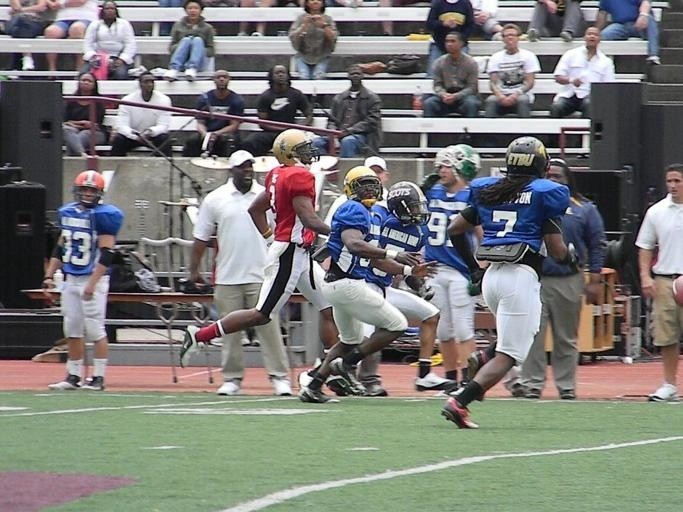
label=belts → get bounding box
[654,274,680,279]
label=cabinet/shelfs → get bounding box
[546,268,615,363]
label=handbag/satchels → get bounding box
[386,54,421,74]
[9,10,44,37]
[355,61,386,74]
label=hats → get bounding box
[364,155,386,172]
[228,149,255,170]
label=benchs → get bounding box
[0,0,668,157]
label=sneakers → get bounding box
[559,389,575,400]
[46,374,82,390]
[178,325,205,368]
[559,31,572,42]
[645,55,661,65]
[81,376,104,392]
[647,383,680,405]
[22,56,35,70]
[527,28,535,42]
[216,382,239,396]
[440,397,479,429]
[414,350,541,402]
[274,380,292,396]
[296,356,387,404]
[183,67,196,81]
[162,69,178,81]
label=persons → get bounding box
[0,0,682,428]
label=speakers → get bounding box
[568,82,683,275]
[0,80,62,208]
[0,182,47,309]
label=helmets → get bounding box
[272,128,320,167]
[504,136,550,179]
[386,180,431,226]
[433,143,481,182]
[71,170,104,205]
[342,165,383,209]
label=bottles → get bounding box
[413,85,423,110]
[56,267,62,288]
